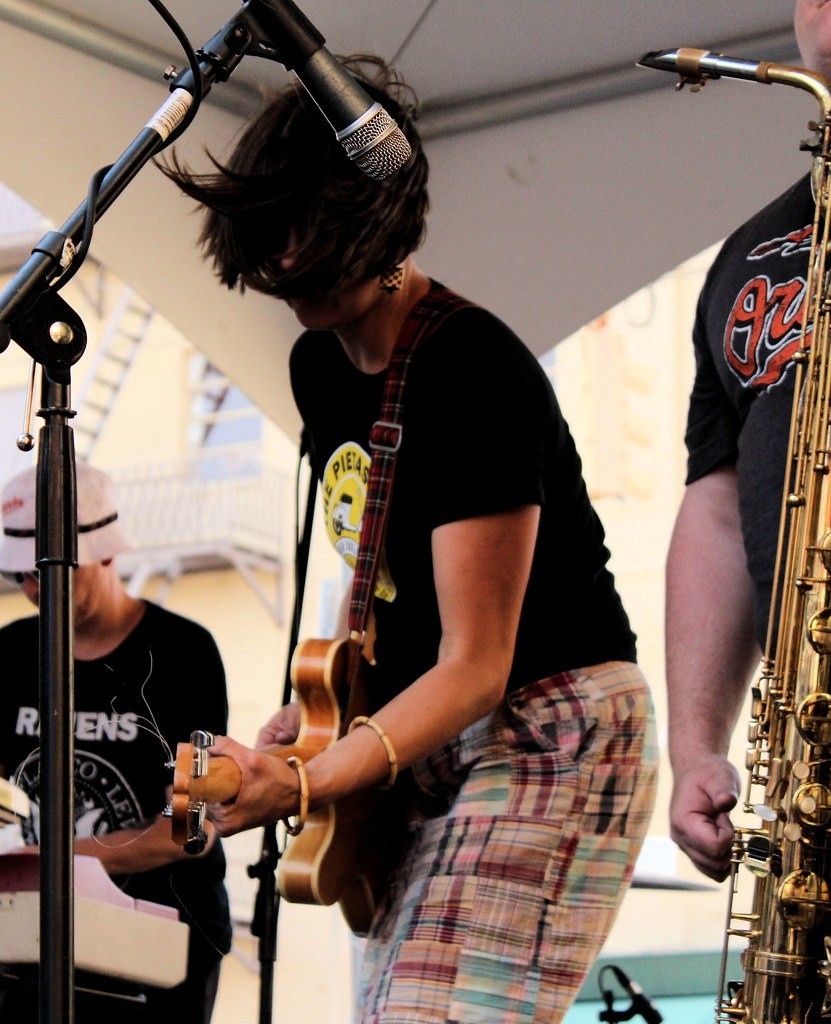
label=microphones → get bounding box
[611,964,663,1024]
[242,0,412,180]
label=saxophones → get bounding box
[636,45,831,1024]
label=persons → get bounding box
[664,1,830,883]
[146,52,658,1024]
[1,461,233,1024]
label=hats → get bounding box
[0,465,125,574]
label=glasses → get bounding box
[13,570,40,584]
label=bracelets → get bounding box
[347,716,398,791]
[282,757,309,836]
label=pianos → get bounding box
[0,779,198,1005]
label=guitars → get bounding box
[167,635,388,937]
